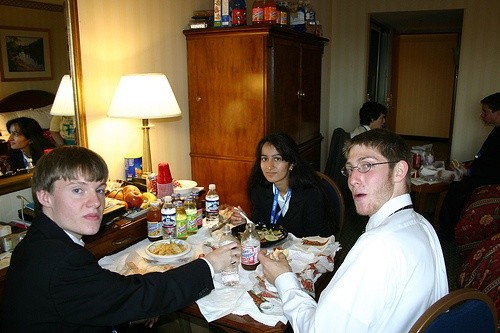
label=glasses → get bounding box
[341,159,400,177]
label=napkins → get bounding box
[410,178,442,186]
[195,287,246,323]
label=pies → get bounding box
[450,159,460,169]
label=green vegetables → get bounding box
[254,227,282,235]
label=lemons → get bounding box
[264,235,278,241]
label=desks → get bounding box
[407,159,470,233]
[98,217,343,333]
[0,190,208,302]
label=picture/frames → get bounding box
[0,26,54,82]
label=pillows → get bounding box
[0,103,54,142]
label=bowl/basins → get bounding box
[173,179,197,194]
[231,223,288,248]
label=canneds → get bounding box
[413,153,422,169]
[146,176,157,196]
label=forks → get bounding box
[234,207,259,226]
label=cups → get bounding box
[156,162,173,199]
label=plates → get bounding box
[145,239,192,258]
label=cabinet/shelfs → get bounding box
[182,23,330,230]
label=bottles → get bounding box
[251,0,315,33]
[205,184,220,225]
[184,197,198,236]
[241,223,261,271]
[190,192,202,229]
[218,225,239,286]
[231,0,247,27]
[146,193,188,241]
[425,147,434,166]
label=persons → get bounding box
[257,128,449,333]
[0,147,241,333]
[230,133,336,237]
[50,115,76,145]
[350,101,388,140]
[438,92,500,224]
[6,117,44,171]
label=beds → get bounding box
[0,89,59,156]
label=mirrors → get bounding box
[0,0,88,196]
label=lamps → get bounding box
[49,75,76,116]
[107,73,183,180]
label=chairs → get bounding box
[409,287,498,333]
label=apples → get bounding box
[108,185,144,208]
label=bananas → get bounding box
[139,192,157,209]
